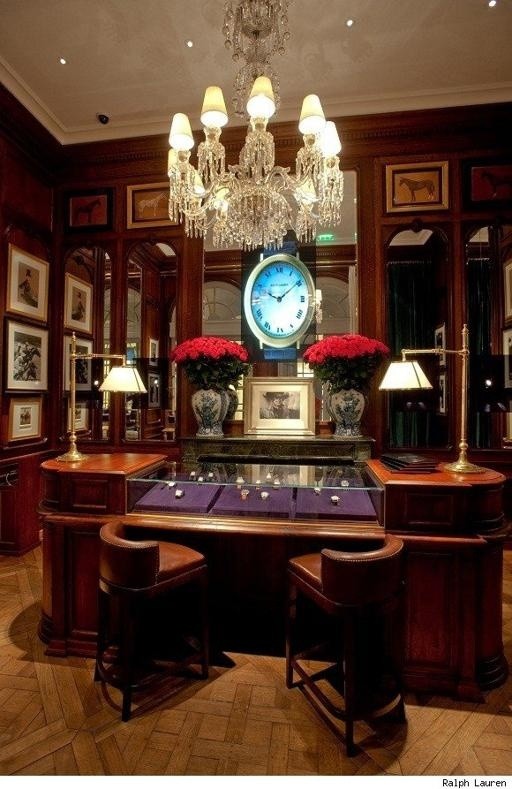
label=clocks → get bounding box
[242,251,322,353]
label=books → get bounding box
[377,452,438,474]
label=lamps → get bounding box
[164,1,346,252]
[376,321,483,477]
[53,327,150,466]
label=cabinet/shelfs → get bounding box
[39,447,508,705]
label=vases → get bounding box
[327,388,373,438]
[192,385,229,438]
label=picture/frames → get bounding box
[62,270,94,435]
[501,259,512,327]
[460,155,511,213]
[432,322,447,372]
[147,336,160,368]
[6,242,50,323]
[381,158,452,218]
[436,371,448,417]
[241,375,316,438]
[3,314,50,399]
[9,397,46,443]
[126,182,180,231]
[500,327,512,391]
[146,370,161,409]
[64,184,116,234]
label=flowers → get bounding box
[301,331,393,395]
[169,335,251,394]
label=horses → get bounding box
[135,191,168,218]
[74,199,101,225]
[22,347,41,365]
[398,177,435,201]
[480,171,512,194]
[13,362,38,381]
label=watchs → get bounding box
[167,471,351,506]
[242,238,323,362]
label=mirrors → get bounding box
[388,227,453,451]
[62,242,111,440]
[465,224,512,450]
[127,240,179,441]
[198,171,357,344]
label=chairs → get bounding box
[161,410,176,441]
[282,533,416,762]
[89,516,215,722]
